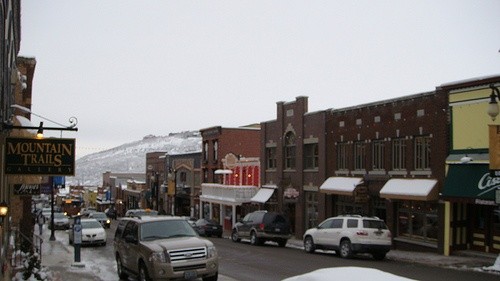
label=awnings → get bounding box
[10,114,50,185]
[379,178,439,201]
[251,187,277,203]
[319,176,365,196]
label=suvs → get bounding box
[230,209,293,248]
[42,208,51,219]
[113,213,218,281]
[303,214,393,258]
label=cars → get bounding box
[88,212,110,228]
[68,218,107,247]
[182,216,196,226]
[125,208,158,215]
[72,206,96,223]
[46,213,70,230]
[191,218,223,237]
